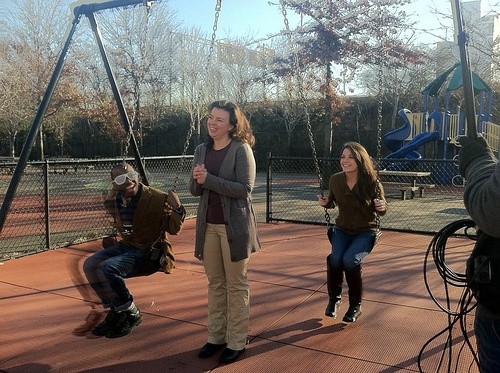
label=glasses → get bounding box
[110,172,140,185]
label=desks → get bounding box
[48,158,94,174]
[377,170,431,199]
[0,163,30,175]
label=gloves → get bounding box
[453,132,492,178]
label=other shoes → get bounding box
[105,308,143,337]
[198,343,227,359]
[219,348,246,364]
[325,299,341,317]
[91,310,122,337]
[343,305,361,322]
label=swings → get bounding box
[278,0,385,250]
[111,1,222,274]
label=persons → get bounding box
[187,99,262,363]
[82,162,186,338]
[458,130,500,373]
[319,142,388,322]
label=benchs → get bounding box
[380,182,436,189]
[380,187,419,191]
[50,165,94,170]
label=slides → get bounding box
[381,107,441,170]
